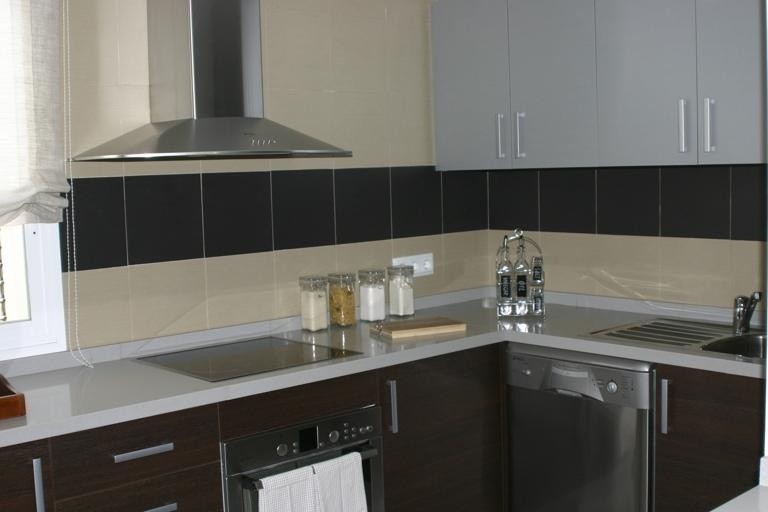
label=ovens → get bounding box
[218,404,387,512]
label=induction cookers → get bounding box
[128,335,366,384]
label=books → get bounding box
[371,315,468,339]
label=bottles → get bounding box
[296,264,417,334]
[495,235,544,315]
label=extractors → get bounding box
[64,1,354,164]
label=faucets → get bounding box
[734,291,762,333]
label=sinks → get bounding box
[700,333,766,364]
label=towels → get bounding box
[257,452,370,511]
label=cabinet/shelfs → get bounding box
[52,403,223,511]
[0,439,50,512]
[381,340,508,512]
[429,1,596,173]
[595,1,765,170]
[650,364,765,512]
